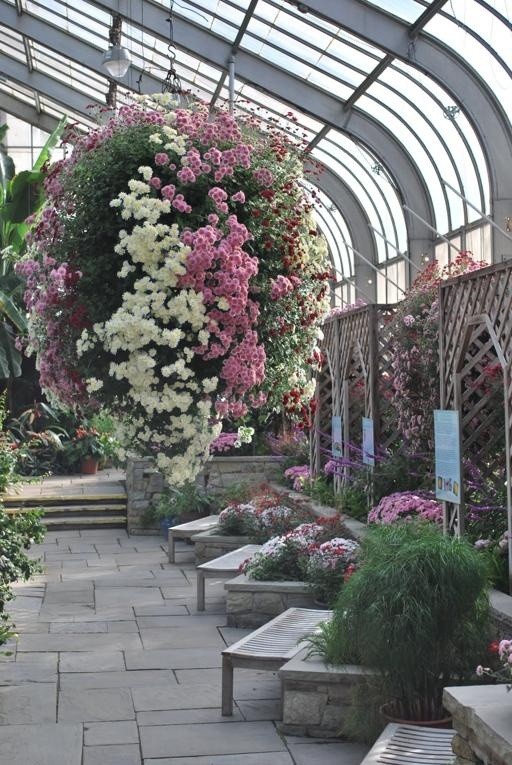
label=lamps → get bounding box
[102,40,134,78]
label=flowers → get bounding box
[11,97,340,489]
[306,534,364,598]
[70,419,107,460]
[474,634,512,683]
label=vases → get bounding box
[314,599,334,612]
[79,458,98,475]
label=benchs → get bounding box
[360,721,460,765]
[195,544,263,611]
[165,510,216,564]
[220,604,337,720]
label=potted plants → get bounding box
[137,482,222,538]
[295,520,504,747]
